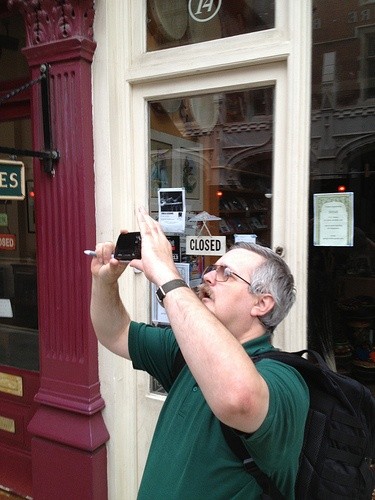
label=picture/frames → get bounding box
[150,128,178,212]
[178,137,203,212]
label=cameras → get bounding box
[114,231,141,260]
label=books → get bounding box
[219,185,270,247]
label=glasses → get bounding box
[202,265,266,296]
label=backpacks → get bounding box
[218,349,375,500]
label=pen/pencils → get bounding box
[82,249,112,264]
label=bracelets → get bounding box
[155,278,190,308]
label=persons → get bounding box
[90,209,311,499]
